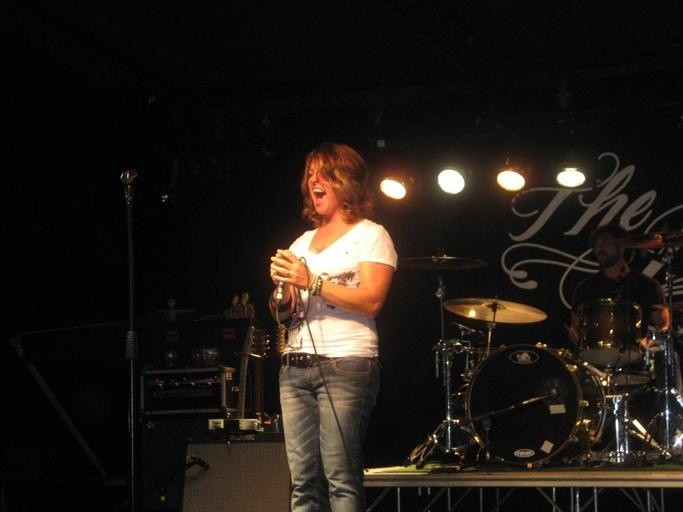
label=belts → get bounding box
[279,352,316,368]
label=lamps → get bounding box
[379,165,587,200]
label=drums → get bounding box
[608,326,658,392]
[464,342,609,465]
[617,385,683,459]
[577,297,646,367]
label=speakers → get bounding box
[138,412,236,512]
[177,429,293,512]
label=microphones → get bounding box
[547,387,560,401]
[190,454,209,469]
[457,324,484,336]
[276,248,295,304]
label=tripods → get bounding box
[601,258,682,463]
[403,272,474,469]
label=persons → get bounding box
[269,142,398,512]
[567,224,681,463]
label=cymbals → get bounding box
[442,297,548,323]
[399,255,488,273]
[612,230,683,249]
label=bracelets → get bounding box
[310,275,322,297]
